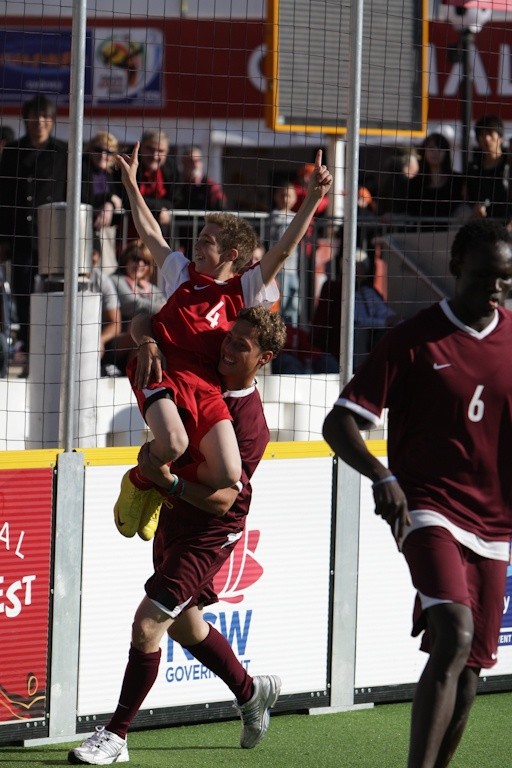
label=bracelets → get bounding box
[137,339,157,353]
[371,475,396,489]
[163,473,185,499]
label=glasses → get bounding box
[94,146,116,157]
[132,255,153,266]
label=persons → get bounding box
[65,303,287,765]
[0,94,512,379]
[114,139,333,541]
[320,217,512,768]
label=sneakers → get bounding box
[113,469,154,537]
[233,674,282,749]
[139,488,173,542]
[68,724,130,766]
